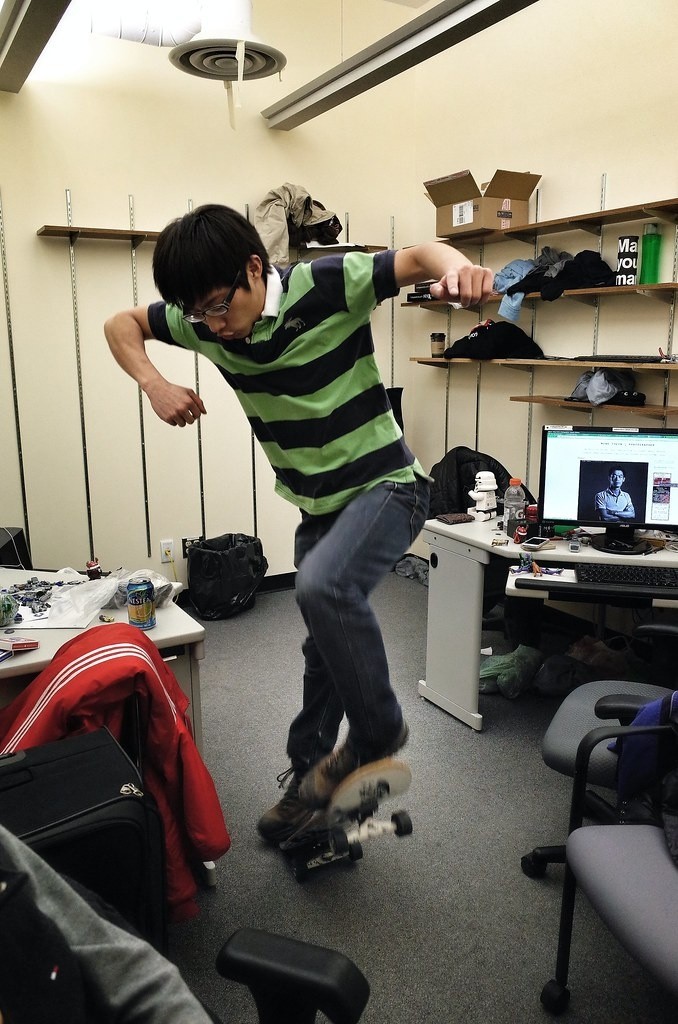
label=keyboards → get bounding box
[574,562,678,587]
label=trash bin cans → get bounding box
[189,534,260,620]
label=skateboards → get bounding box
[279,756,414,888]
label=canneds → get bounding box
[126,577,157,630]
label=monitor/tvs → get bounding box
[538,425,678,555]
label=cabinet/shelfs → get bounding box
[402,198,678,417]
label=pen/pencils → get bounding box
[549,538,568,541]
[643,546,664,556]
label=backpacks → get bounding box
[0,870,90,1024]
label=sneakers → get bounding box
[298,721,408,806]
[257,758,318,840]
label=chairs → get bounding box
[521,622,678,1012]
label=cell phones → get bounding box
[521,537,550,549]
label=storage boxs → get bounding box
[422,168,541,237]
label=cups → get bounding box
[430,332,446,358]
[615,236,639,286]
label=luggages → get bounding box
[0,728,169,960]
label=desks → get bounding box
[1,603,204,763]
[419,517,678,730]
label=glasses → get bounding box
[182,270,241,323]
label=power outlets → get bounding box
[159,536,203,562]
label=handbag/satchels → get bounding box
[479,635,634,700]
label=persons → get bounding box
[104,204,493,842]
[594,466,636,523]
[0,826,223,1024]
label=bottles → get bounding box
[502,478,525,533]
[638,222,663,285]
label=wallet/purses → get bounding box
[435,513,475,525]
